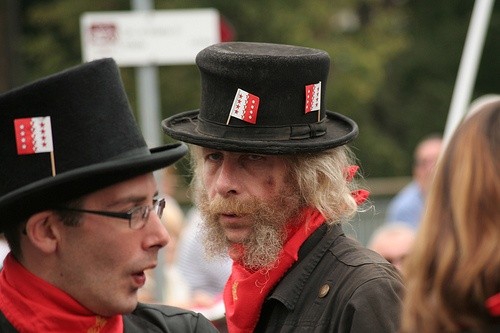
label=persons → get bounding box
[387,137,447,230]
[366,219,413,276]
[400,98,500,333]
[0,55,222,333]
[135,164,233,320]
[160,42,406,333]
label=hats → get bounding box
[0,58,188,217]
[161,40,358,154]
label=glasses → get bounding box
[26,198,164,230]
